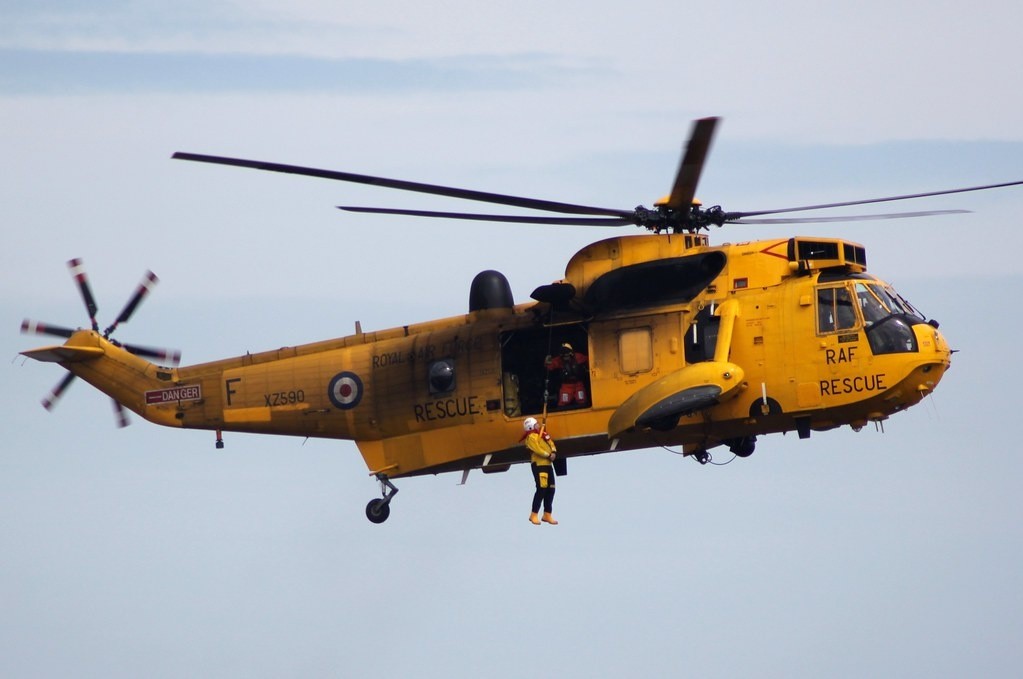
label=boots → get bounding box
[541,512,558,524]
[529,512,541,524]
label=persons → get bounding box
[545,342,588,406]
[524,417,558,525]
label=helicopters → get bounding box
[13,115,1023,526]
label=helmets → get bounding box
[562,343,572,350]
[524,417,537,432]
